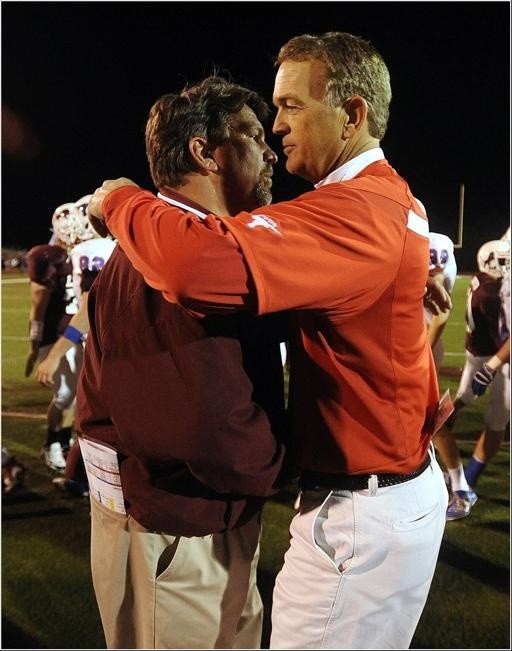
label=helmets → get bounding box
[477,240,512,279]
[51,201,77,246]
[74,194,101,240]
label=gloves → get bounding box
[471,354,503,396]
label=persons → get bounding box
[86,32,451,650]
[1,194,510,520]
[70,77,300,651]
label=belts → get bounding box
[298,450,432,492]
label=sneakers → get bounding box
[40,440,67,474]
[446,485,478,522]
[1,458,25,497]
